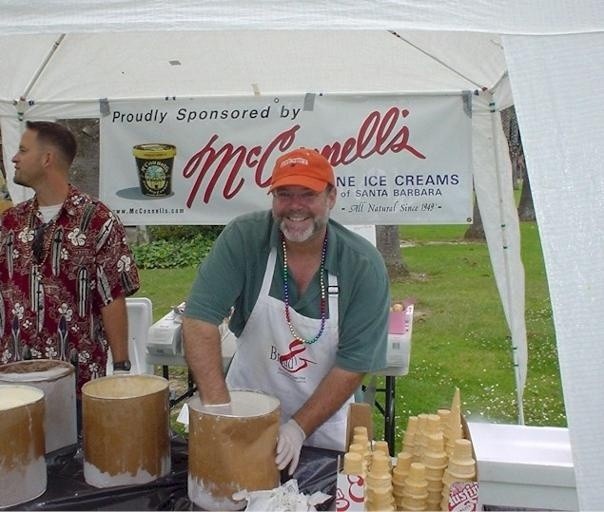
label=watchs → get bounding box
[113,360,132,371]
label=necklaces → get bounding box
[282,236,328,345]
[24,210,57,268]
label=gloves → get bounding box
[274,418,306,475]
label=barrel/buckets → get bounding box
[79,372,173,491]
[0,384,48,510]
[184,388,284,511]
[0,359,77,455]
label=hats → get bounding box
[266,149,335,195]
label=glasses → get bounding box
[31,223,44,250]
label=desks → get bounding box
[147,311,409,455]
[1,435,393,509]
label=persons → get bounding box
[183,148,391,476]
[1,120,140,438]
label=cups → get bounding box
[130,144,176,198]
